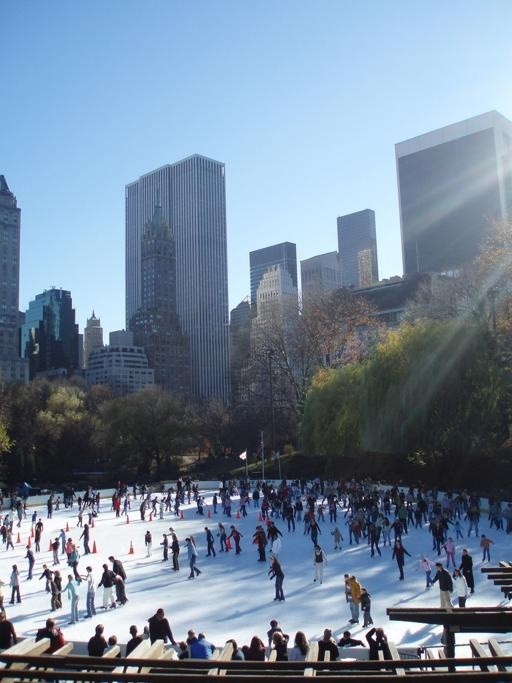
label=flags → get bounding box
[239,451,247,460]
